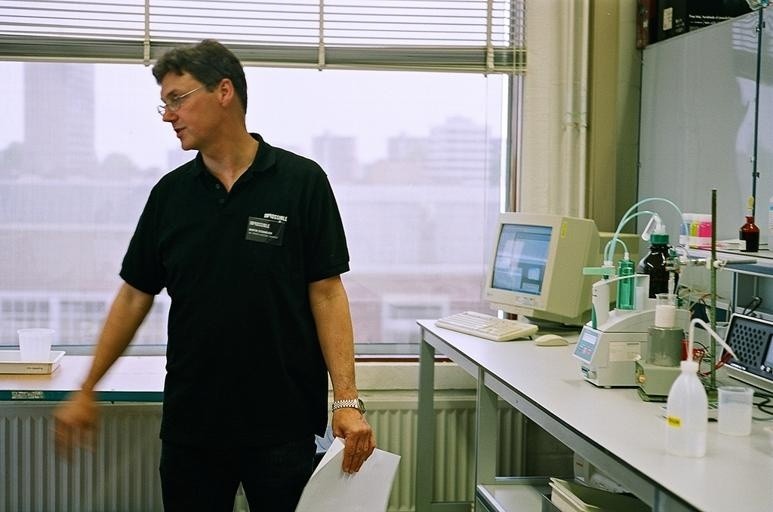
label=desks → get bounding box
[413,316,772,510]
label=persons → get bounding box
[54,38,376,512]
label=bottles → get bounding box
[616,258,635,310]
[666,360,709,458]
[767,195,773,250]
[740,196,759,252]
[679,212,712,249]
[642,233,681,299]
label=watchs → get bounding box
[332,397,367,414]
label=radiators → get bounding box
[0,395,527,512]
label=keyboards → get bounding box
[437,311,537,341]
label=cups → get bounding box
[16,330,51,360]
[653,292,677,327]
[719,387,754,438]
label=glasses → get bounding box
[157,76,232,117]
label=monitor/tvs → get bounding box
[486,208,620,329]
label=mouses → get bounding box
[536,334,568,345]
